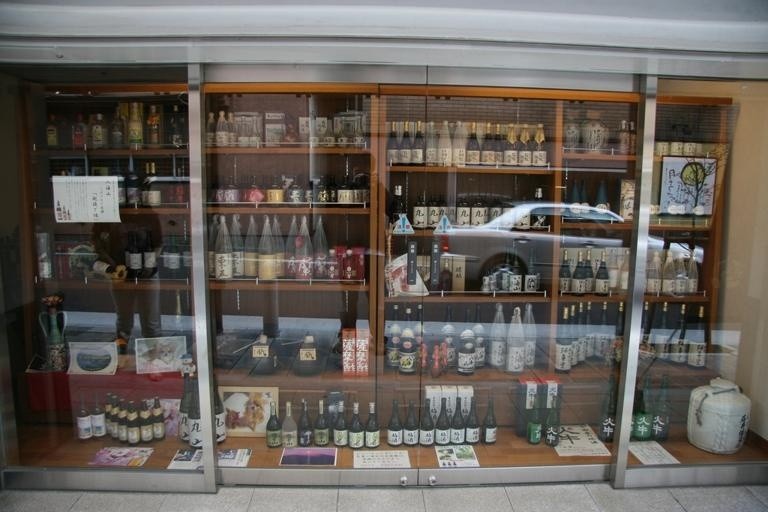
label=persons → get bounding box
[92,171,163,354]
[320,152,402,355]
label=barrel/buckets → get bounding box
[689,378,751,452]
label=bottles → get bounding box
[73,390,168,446]
[439,257,453,293]
[659,117,710,157]
[212,376,230,447]
[601,372,672,441]
[204,154,368,288]
[527,394,561,446]
[643,303,704,369]
[207,109,263,148]
[280,118,300,146]
[387,119,549,168]
[385,172,611,230]
[615,116,636,156]
[564,109,609,154]
[33,95,191,282]
[386,305,540,374]
[479,229,700,297]
[560,303,622,367]
[385,396,500,445]
[265,395,381,451]
[308,109,366,150]
[177,374,200,448]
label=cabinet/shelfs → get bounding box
[1,81,767,488]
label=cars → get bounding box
[432,204,705,292]
